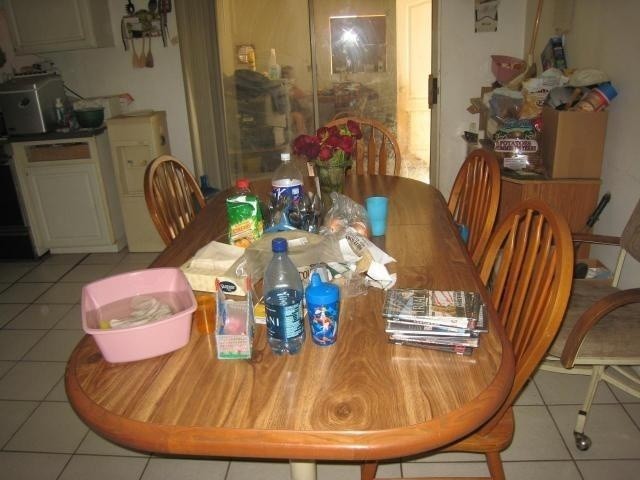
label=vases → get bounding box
[316,162,344,214]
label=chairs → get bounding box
[447,148,500,272]
[317,117,401,179]
[142,155,207,250]
[548,198,640,451]
[358,197,575,480]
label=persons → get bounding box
[282,64,311,135]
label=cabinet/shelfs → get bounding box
[1,124,128,262]
[0,0,114,57]
[496,175,599,259]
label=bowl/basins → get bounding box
[80,266,199,364]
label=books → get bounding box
[384,288,490,355]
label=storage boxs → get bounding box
[541,100,611,180]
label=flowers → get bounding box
[294,119,362,194]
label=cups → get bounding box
[194,298,217,335]
[364,195,390,237]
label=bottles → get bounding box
[268,48,281,81]
[305,273,341,347]
[263,237,306,357]
[270,153,305,233]
[225,178,261,247]
[55,97,65,123]
